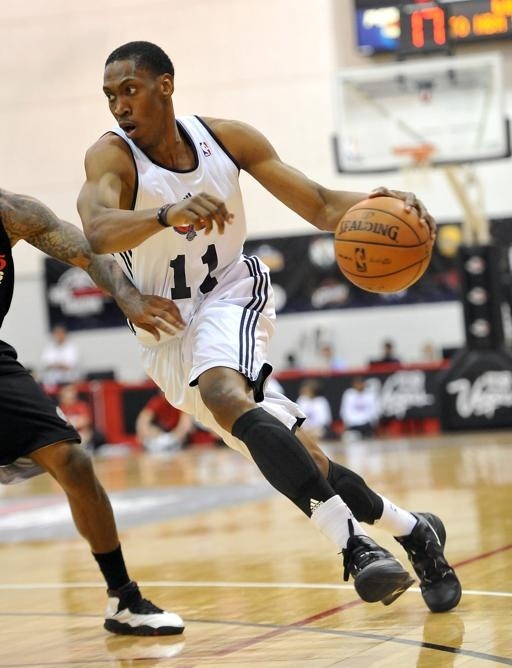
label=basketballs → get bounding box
[335,196,433,294]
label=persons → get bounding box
[33,321,199,456]
[278,330,456,443]
[0,188,192,638]
[77,40,461,614]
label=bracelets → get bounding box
[157,203,174,227]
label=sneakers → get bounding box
[397,511,461,613]
[104,580,184,636]
[342,518,415,605]
